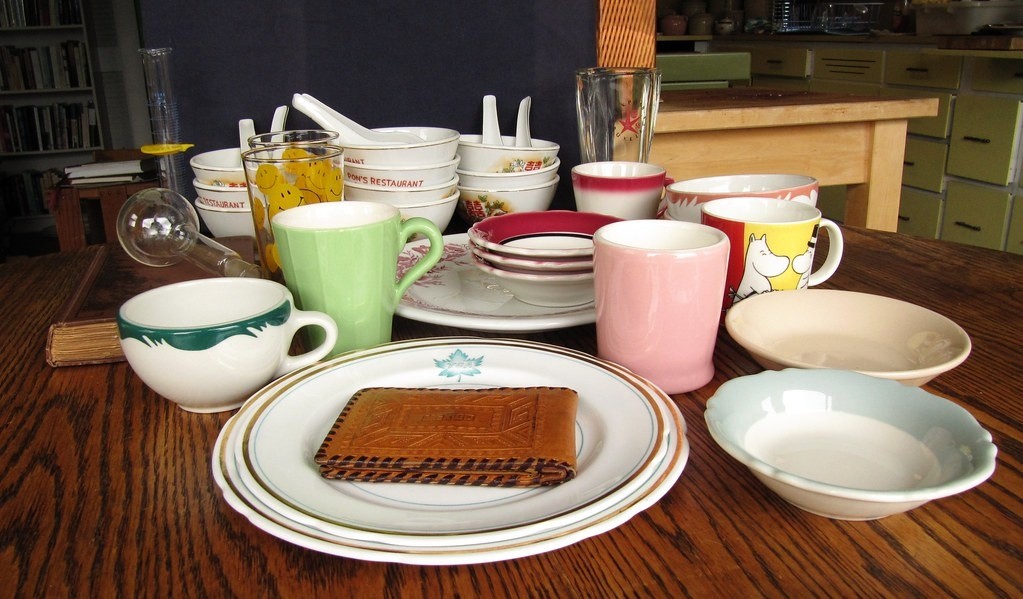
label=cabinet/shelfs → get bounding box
[714,39,1023,254]
[0,0,106,244]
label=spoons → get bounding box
[291,91,425,146]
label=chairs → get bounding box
[91,149,155,163]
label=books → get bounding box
[0,0,104,218]
[63,156,155,184]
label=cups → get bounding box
[575,66,662,165]
[116,276,337,413]
[271,200,444,361]
[701,197,844,328]
[593,219,731,396]
[571,161,676,220]
[242,131,345,281]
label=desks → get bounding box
[0,222,1023,599]
[48,180,160,251]
[651,86,941,234]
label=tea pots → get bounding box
[661,11,688,35]
[689,10,713,35]
[714,17,739,34]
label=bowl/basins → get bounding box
[190,147,317,261]
[456,133,560,225]
[327,127,461,243]
[664,175,819,224]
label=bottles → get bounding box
[116,187,271,281]
[892,0,902,33]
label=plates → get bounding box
[211,335,689,567]
[703,368,998,520]
[395,232,599,333]
[467,210,624,307]
[724,290,972,388]
